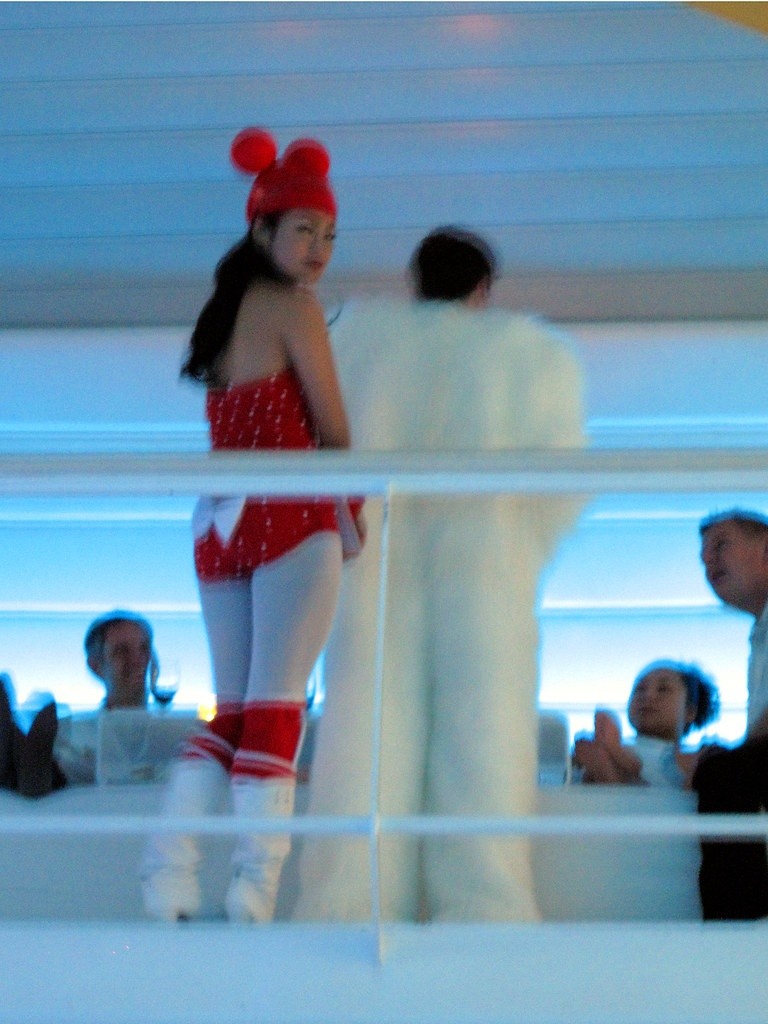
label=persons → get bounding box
[564,507,768,927]
[0,608,178,800]
[138,129,369,930]
[293,227,593,927]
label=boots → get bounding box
[223,783,295,925]
[141,759,227,926]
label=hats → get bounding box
[230,127,337,227]
